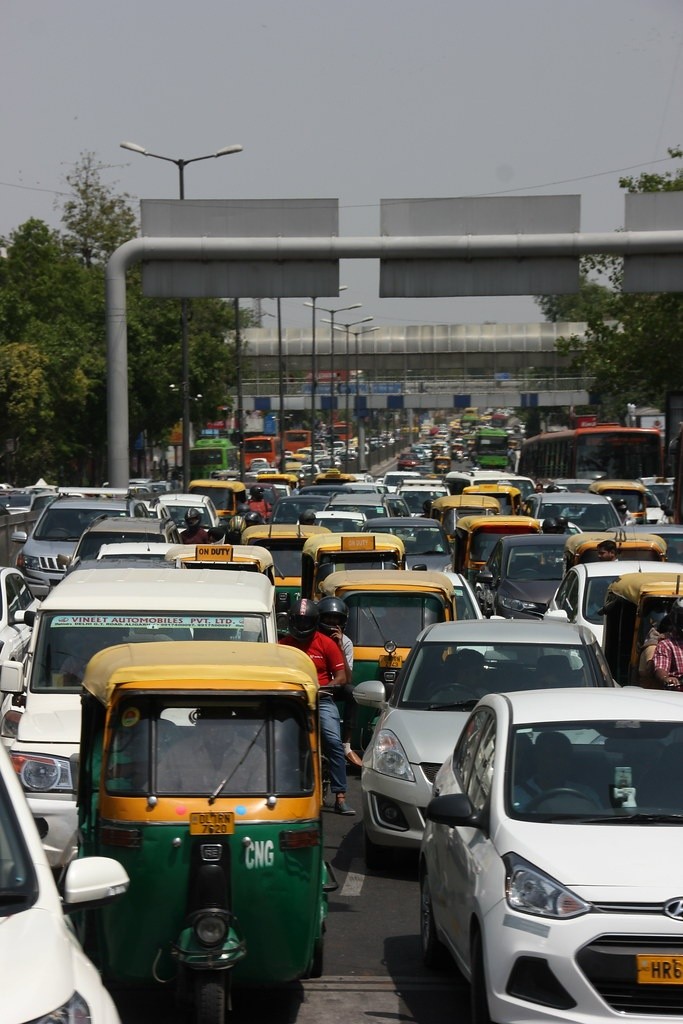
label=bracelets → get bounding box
[664,676,667,677]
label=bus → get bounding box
[326,421,354,439]
[474,428,511,469]
[236,437,282,473]
[284,430,311,452]
[188,438,240,481]
[517,427,664,482]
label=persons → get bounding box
[278,595,362,815]
[429,649,491,704]
[535,483,560,493]
[413,500,440,531]
[82,699,273,833]
[59,636,104,681]
[597,540,618,561]
[561,504,586,518]
[543,518,562,534]
[180,485,273,544]
[512,731,604,814]
[299,511,316,525]
[639,596,683,687]
[293,478,305,494]
[615,497,636,525]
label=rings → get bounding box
[669,680,671,682]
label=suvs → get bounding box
[8,491,150,599]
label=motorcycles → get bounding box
[434,455,453,474]
[71,641,326,1024]
[565,531,668,570]
[316,568,458,768]
[188,480,247,523]
[597,571,682,688]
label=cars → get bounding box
[541,562,682,671]
[424,687,683,1023]
[352,619,643,847]
[396,452,421,471]
[475,533,572,622]
[0,409,683,619]
[2,568,40,712]
[0,567,278,874]
[1,743,130,1024]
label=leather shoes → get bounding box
[344,750,363,767]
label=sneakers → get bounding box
[334,801,356,814]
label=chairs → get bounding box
[531,654,578,689]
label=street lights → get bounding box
[302,285,380,480]
[120,138,244,499]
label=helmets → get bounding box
[247,485,265,499]
[184,508,201,529]
[288,598,319,640]
[547,486,559,493]
[316,596,349,634]
[614,497,627,510]
[542,516,569,529]
[245,512,260,523]
[423,499,434,512]
[237,505,249,512]
[299,511,316,525]
[229,516,246,532]
